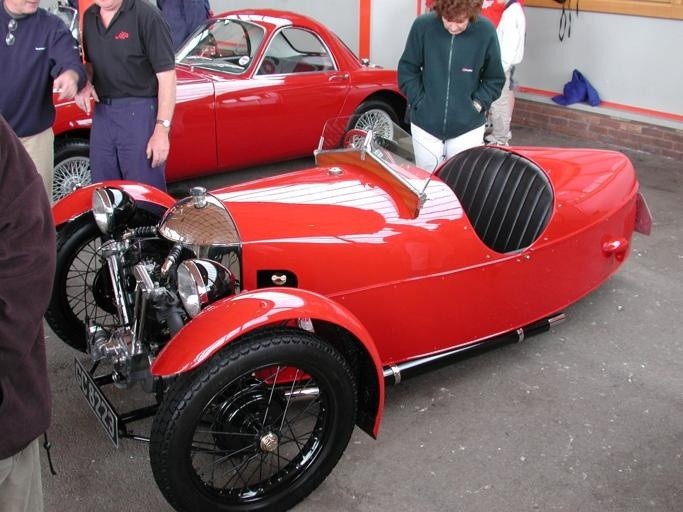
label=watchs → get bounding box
[154,119,171,128]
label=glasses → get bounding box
[6,19,18,46]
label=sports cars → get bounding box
[53,8,416,207]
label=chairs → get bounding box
[436,146,553,253]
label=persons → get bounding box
[73,0,178,194]
[0,0,85,207]
[397,0,507,174]
[157,0,216,56]
[0,115,56,511]
[478,0,525,148]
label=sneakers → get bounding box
[485,131,512,149]
